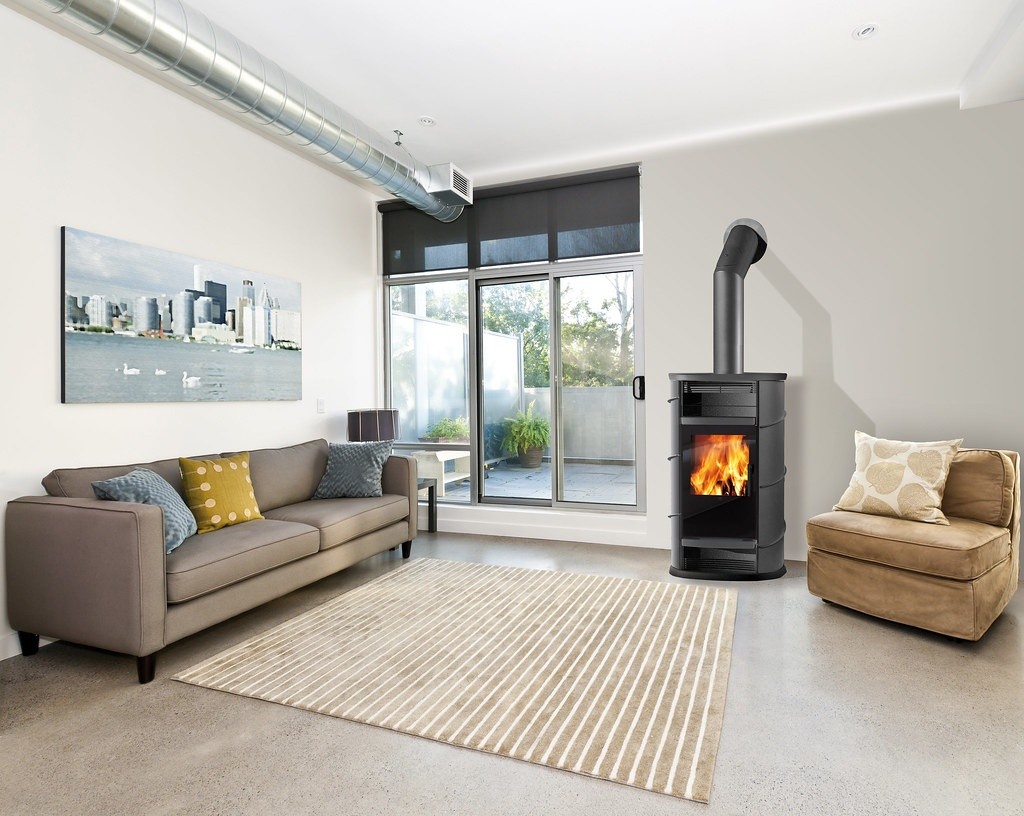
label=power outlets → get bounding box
[317,399,325,412]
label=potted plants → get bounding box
[497,398,551,467]
[417,415,470,451]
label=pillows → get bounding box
[310,439,395,500]
[832,430,964,525]
[178,451,265,535]
[91,466,198,554]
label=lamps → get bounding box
[346,408,401,442]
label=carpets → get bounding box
[170,557,741,804]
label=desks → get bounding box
[411,451,470,497]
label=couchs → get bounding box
[6,438,418,685]
[805,448,1022,641]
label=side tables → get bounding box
[389,478,437,552]
